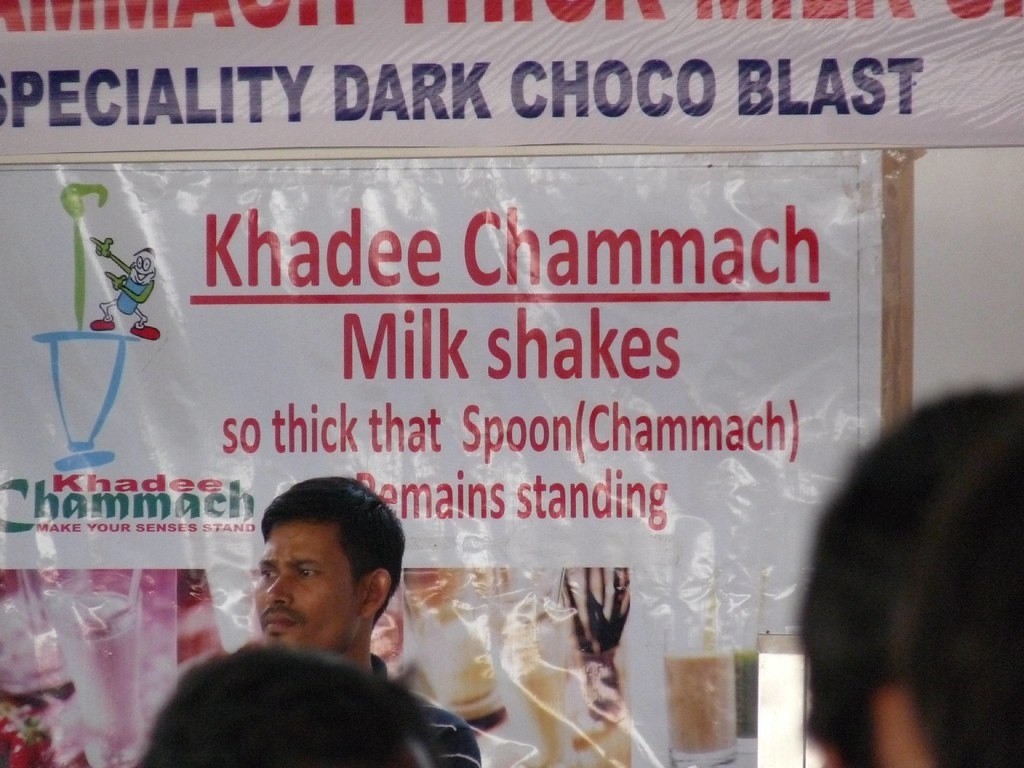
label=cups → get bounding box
[402,568,508,732]
[664,633,737,764]
[44,573,143,767]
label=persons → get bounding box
[801,389,1024,768]
[145,645,440,768]
[255,478,481,768]
